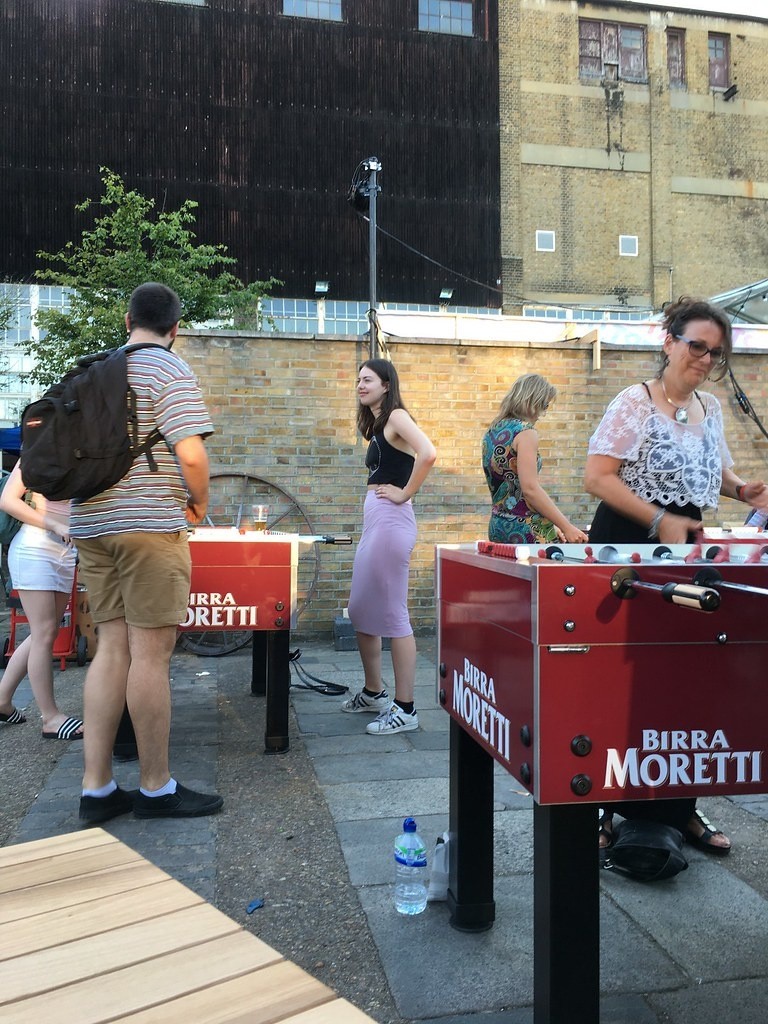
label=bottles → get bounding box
[392,817,430,915]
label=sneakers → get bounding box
[79,785,138,825]
[340,690,389,713]
[133,782,224,819]
[366,700,418,735]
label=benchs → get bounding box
[0,827,378,1024]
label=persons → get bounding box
[583,295,768,860]
[0,458,84,740]
[341,359,437,735]
[69,282,224,821]
[482,374,588,543]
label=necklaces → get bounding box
[662,380,693,424]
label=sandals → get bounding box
[683,809,731,857]
[599,808,614,849]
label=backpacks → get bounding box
[19,343,176,502]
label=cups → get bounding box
[252,504,269,532]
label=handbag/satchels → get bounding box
[600,820,689,883]
[0,469,36,544]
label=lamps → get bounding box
[439,287,454,300]
[314,280,330,294]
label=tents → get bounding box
[0,427,21,478]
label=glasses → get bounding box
[674,334,724,363]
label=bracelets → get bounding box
[648,508,666,539]
[736,482,749,502]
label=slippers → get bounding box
[1,708,27,726]
[42,717,84,740]
